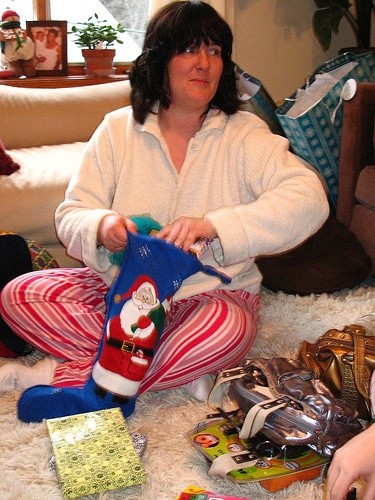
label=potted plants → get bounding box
[68,13,125,76]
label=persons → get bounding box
[0,6,36,77]
[34,31,45,58]
[325,422,375,500]
[0,1,328,420]
[35,28,62,70]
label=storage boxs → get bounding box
[47,407,149,500]
[259,466,323,493]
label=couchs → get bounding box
[0,79,134,278]
[335,85,375,265]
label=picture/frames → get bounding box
[27,21,69,75]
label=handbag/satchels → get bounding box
[274,51,375,208]
[299,321,375,421]
[207,355,362,477]
[231,60,292,151]
